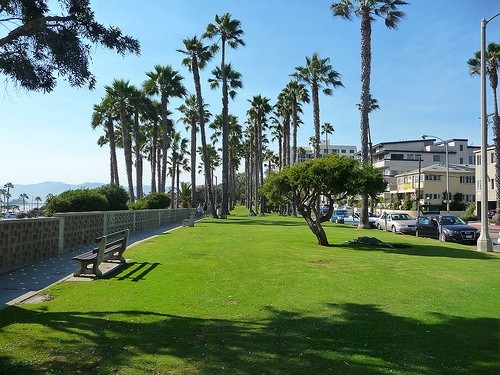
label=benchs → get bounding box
[72,228,130,279]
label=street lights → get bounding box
[421,134,450,216]
[203,173,217,211]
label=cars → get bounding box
[2,211,26,218]
[320,205,381,227]
[415,214,481,245]
[374,212,418,234]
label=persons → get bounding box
[197,202,208,214]
